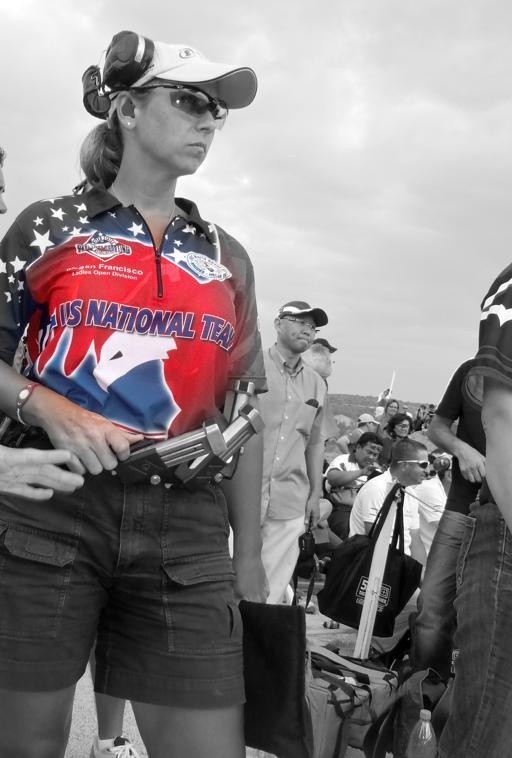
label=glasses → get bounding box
[119,84,228,131]
[398,460,429,469]
[283,316,320,336]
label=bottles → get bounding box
[404,707,439,757]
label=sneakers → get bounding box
[89,736,139,758]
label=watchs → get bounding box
[15,380,41,427]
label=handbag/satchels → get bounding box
[245,639,453,758]
[315,533,422,637]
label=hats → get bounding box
[357,413,380,426]
[96,42,258,129]
[279,301,328,327]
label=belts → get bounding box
[127,457,224,484]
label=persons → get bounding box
[0,29,271,758]
[0,445,90,501]
[0,147,8,214]
[432,263,511,758]
[400,358,485,671]
[85,637,143,758]
[322,388,452,588]
[223,299,337,605]
